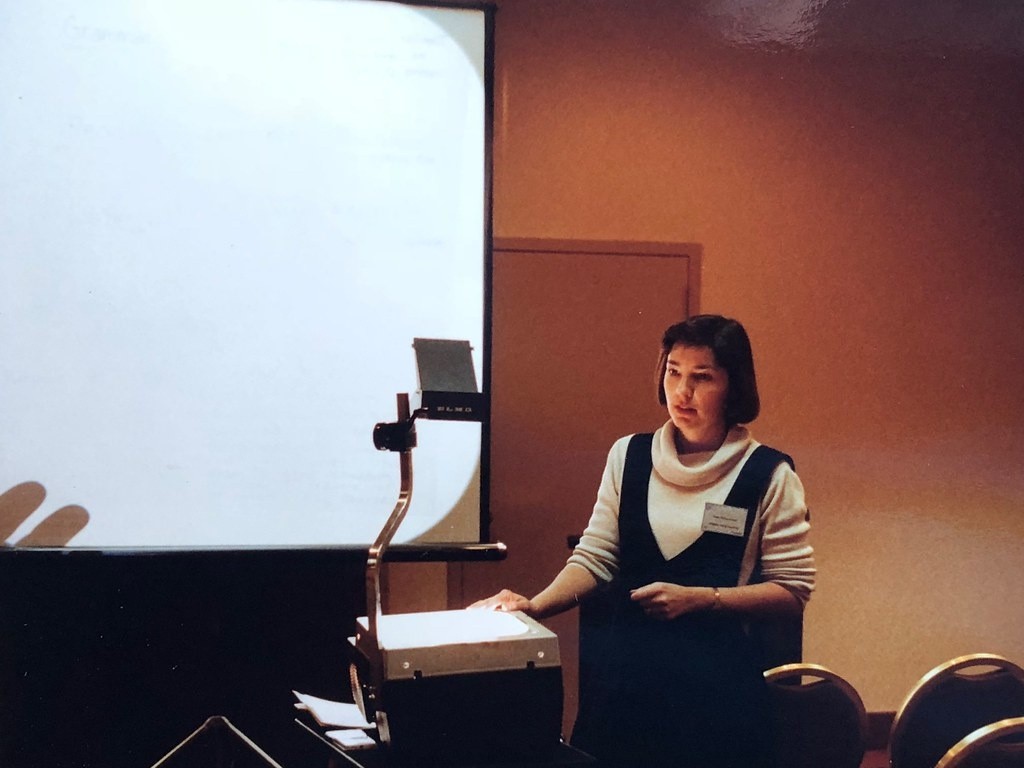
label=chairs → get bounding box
[764,653,1024,768]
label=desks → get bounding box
[295,714,602,768]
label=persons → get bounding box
[466,314,818,768]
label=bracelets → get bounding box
[713,588,720,610]
